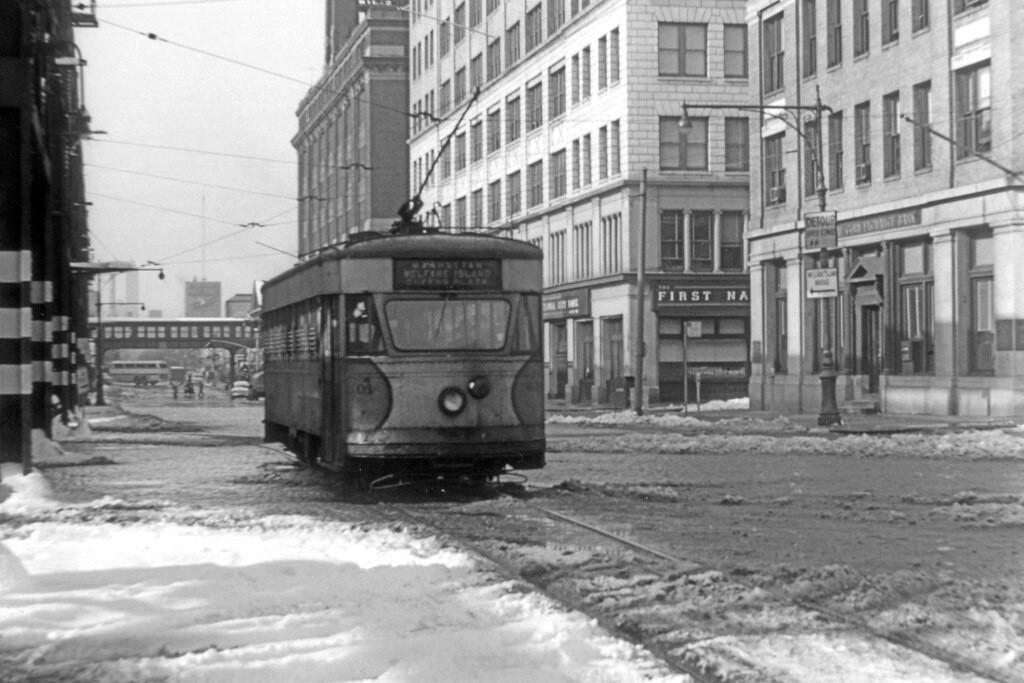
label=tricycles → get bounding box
[184,383,195,395]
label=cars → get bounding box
[230,381,255,400]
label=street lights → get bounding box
[95,266,165,406]
[206,340,251,384]
[678,101,840,426]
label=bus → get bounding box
[108,359,169,386]
[261,218,546,492]
